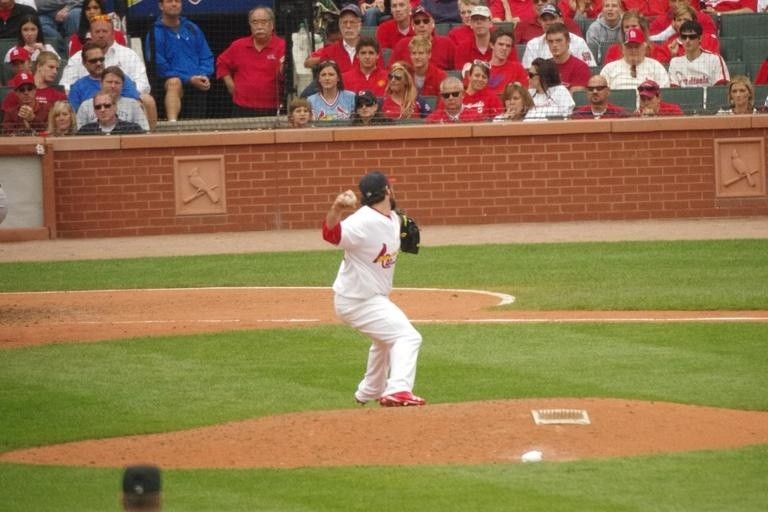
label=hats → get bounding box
[355,90,376,101]
[624,29,645,45]
[412,5,432,18]
[470,5,491,17]
[340,4,361,16]
[9,48,35,87]
[639,80,659,97]
[360,172,388,204]
[539,3,557,17]
[124,468,159,493]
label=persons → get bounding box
[321,172,427,406]
[119,464,162,511]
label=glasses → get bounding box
[441,92,459,97]
[415,19,429,24]
[19,87,32,91]
[586,86,606,90]
[88,58,104,63]
[638,86,655,91]
[389,74,401,80]
[474,59,491,69]
[96,104,110,109]
[320,59,336,66]
[681,34,697,39]
[528,72,539,78]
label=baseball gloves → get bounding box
[400,216,420,253]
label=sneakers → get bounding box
[380,391,425,405]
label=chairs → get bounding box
[322,0,768,117]
[2,23,133,133]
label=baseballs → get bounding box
[345,194,357,206]
